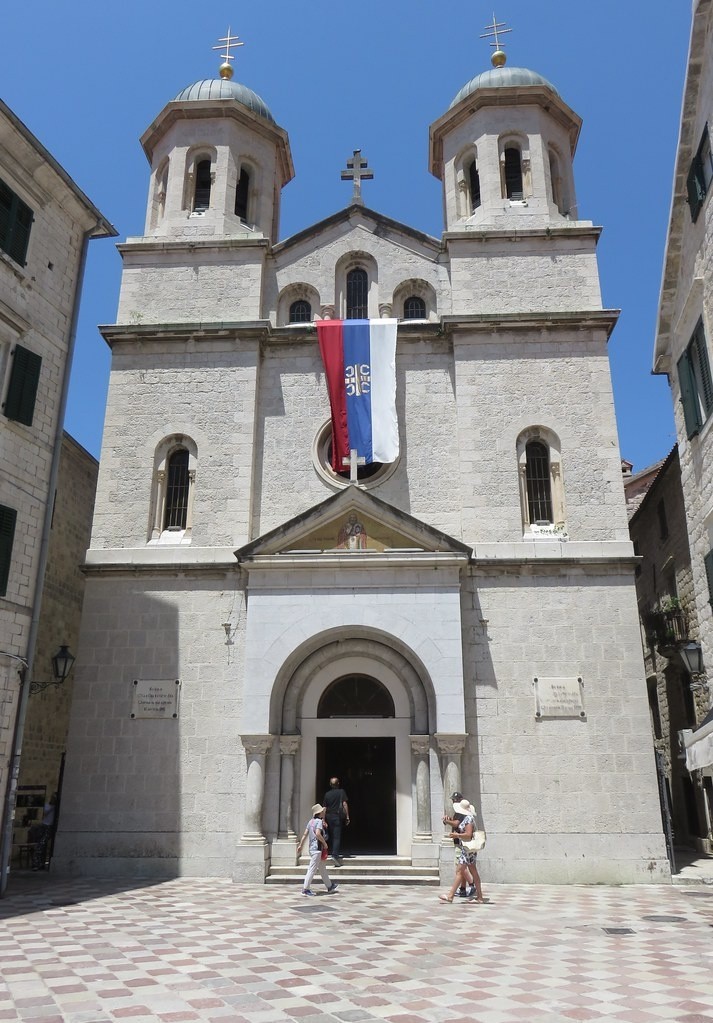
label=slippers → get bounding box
[468,898,484,905]
[438,895,453,903]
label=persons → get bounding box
[296,803,339,897]
[321,777,350,866]
[442,791,476,897]
[437,799,484,904]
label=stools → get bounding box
[14,843,37,867]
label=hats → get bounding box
[450,792,462,800]
[452,799,476,816]
[312,804,327,817]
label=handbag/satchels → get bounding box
[463,831,486,852]
[339,789,347,823]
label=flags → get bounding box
[316,318,399,472]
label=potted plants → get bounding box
[645,598,679,624]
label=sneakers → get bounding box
[328,884,338,893]
[302,889,316,897]
[467,886,476,896]
[454,888,466,897]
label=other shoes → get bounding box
[331,858,340,867]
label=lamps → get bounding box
[679,647,708,693]
[30,646,75,695]
